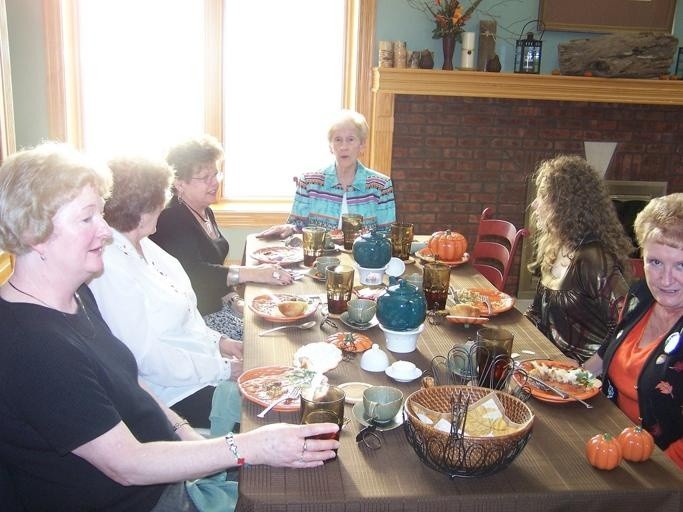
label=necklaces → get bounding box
[179,197,210,223]
[6,277,98,340]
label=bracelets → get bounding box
[226,433,252,472]
[227,294,240,308]
[172,418,190,432]
[229,264,241,286]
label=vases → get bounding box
[442,35,455,70]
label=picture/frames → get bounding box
[537,0,679,37]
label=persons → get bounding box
[523,152,633,380]
[81,155,244,434]
[146,132,295,341]
[254,108,397,238]
[0,140,341,512]
[583,191,683,471]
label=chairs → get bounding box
[628,258,645,281]
[469,207,531,293]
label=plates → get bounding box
[251,246,303,265]
[416,250,471,266]
[248,293,319,323]
[339,312,378,331]
[513,359,599,404]
[447,354,479,381]
[463,288,515,316]
[237,342,422,432]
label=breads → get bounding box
[279,302,308,317]
[449,303,483,318]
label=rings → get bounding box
[272,272,282,280]
[304,440,307,453]
[299,452,305,462]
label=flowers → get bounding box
[407,1,543,51]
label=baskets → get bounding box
[405,385,534,468]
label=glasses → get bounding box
[193,171,223,184]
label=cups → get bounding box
[302,212,377,325]
[453,329,514,389]
[379,41,419,69]
[390,221,415,261]
[423,263,450,312]
[299,386,346,461]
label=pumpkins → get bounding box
[618,424,654,462]
[327,331,373,353]
[428,229,467,260]
[587,433,622,470]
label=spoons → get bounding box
[258,321,316,336]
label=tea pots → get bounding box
[376,275,426,331]
[352,228,391,268]
